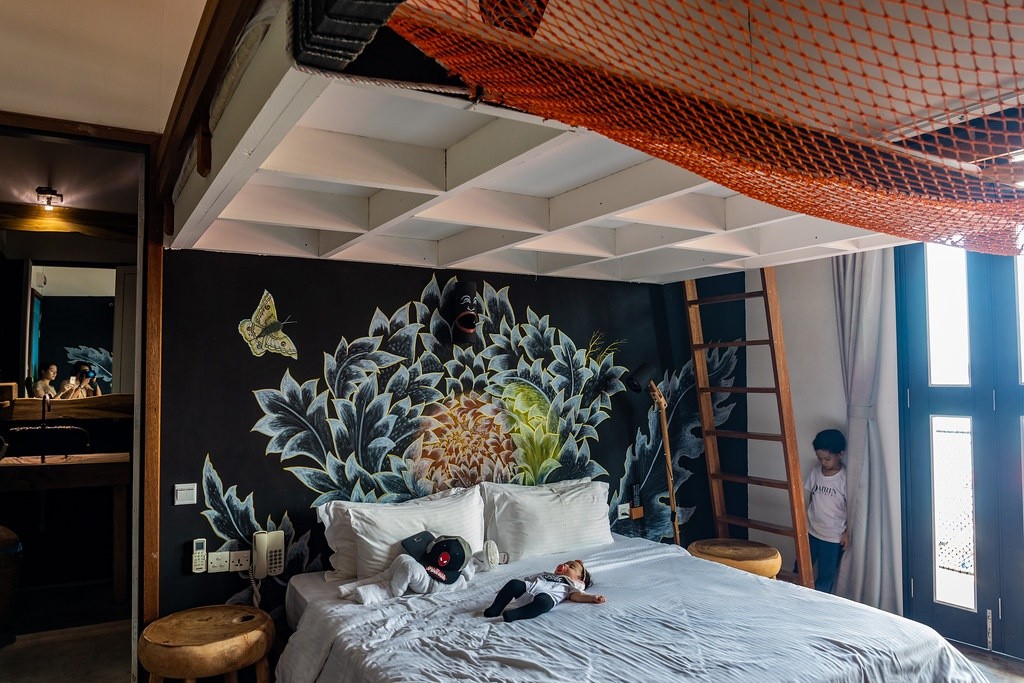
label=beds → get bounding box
[285,529,988,683]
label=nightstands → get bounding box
[687,537,783,580]
[138,604,276,683]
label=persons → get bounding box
[61,360,103,400]
[787,429,852,594]
[31,361,76,400]
[472,558,606,623]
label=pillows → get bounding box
[315,486,468,583]
[479,475,592,543]
[345,484,485,582]
[484,481,615,563]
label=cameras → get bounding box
[80,369,97,379]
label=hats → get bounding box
[424,535,472,584]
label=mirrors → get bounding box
[19,256,118,402]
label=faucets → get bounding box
[40,392,53,429]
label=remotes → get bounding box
[193,538,207,574]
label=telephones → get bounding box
[253,530,285,580]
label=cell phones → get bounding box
[69,376,77,390]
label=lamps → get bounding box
[35,185,64,212]
[627,361,681,548]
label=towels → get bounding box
[335,555,479,607]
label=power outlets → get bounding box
[229,549,250,572]
[617,502,630,520]
